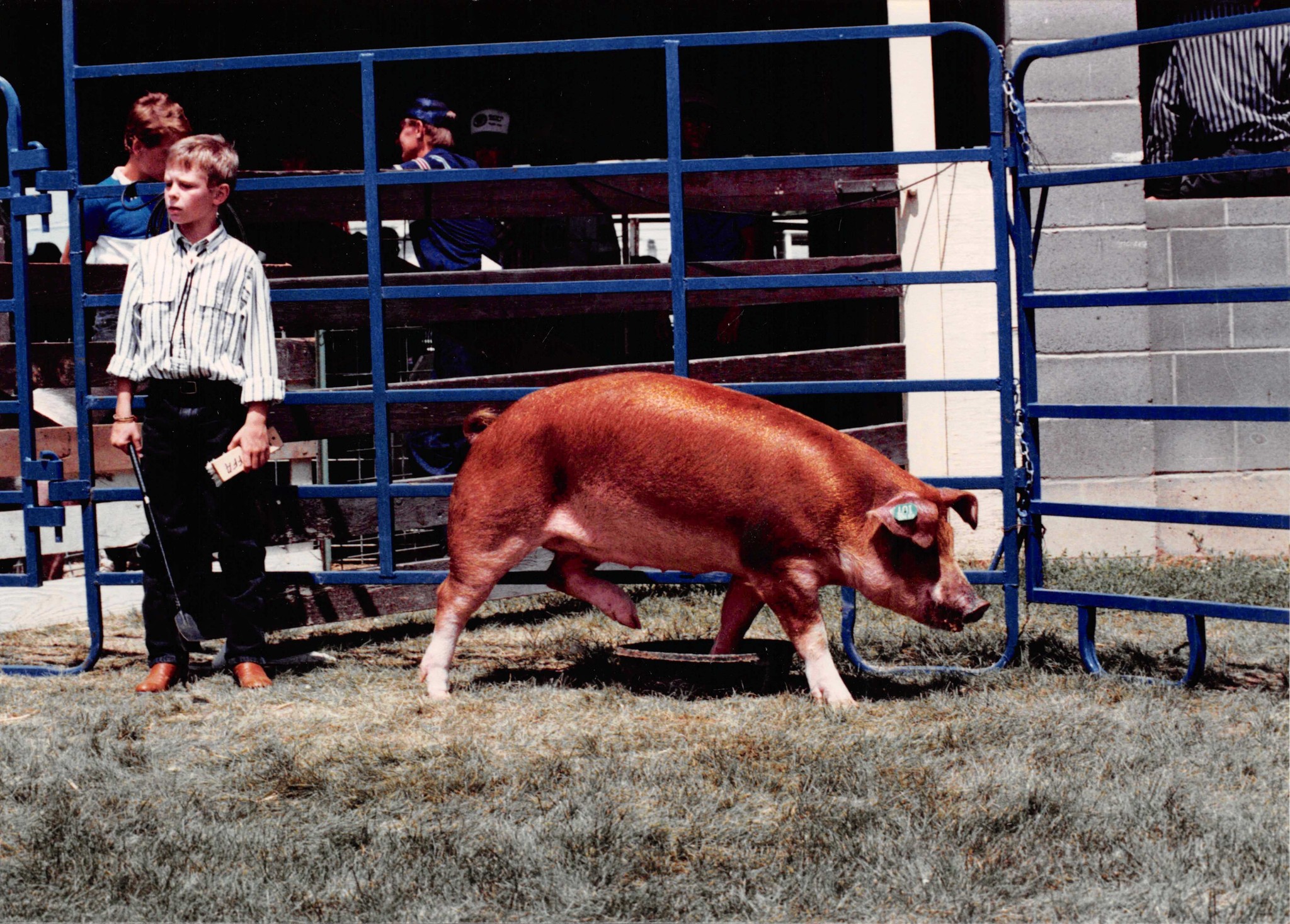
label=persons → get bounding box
[107,135,286,692]
[1145,0,1290,198]
[393,97,757,360]
[59,92,192,385]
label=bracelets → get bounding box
[112,414,137,422]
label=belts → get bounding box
[149,378,240,393]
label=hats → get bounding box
[407,98,449,127]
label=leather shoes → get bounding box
[234,662,272,689]
[135,663,188,692]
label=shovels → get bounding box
[127,442,206,642]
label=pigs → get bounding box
[418,372,992,712]
